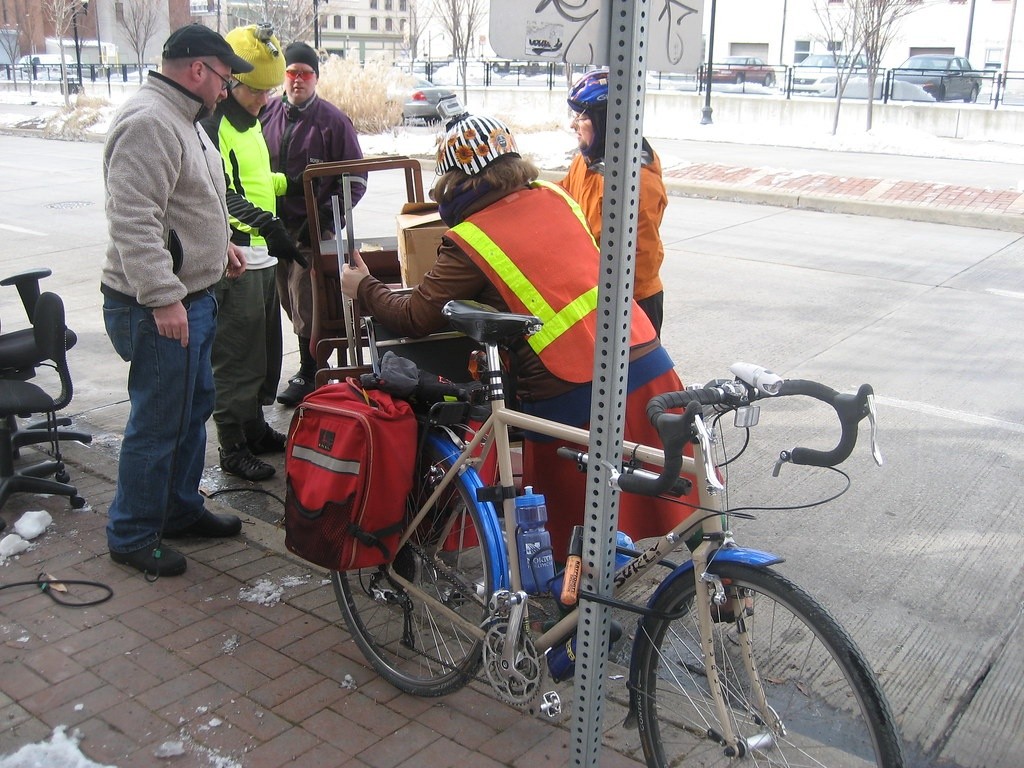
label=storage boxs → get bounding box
[399,198,449,289]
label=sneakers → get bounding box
[277,362,330,405]
[249,427,287,453]
[218,446,276,480]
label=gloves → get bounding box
[361,351,470,411]
[259,216,308,268]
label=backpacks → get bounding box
[282,377,416,570]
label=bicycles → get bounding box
[329,290,908,768]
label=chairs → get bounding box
[922,59,934,68]
[0,291,86,532]
[0,266,92,459]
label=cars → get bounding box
[489,50,551,75]
[698,55,776,87]
[781,52,880,96]
[380,75,455,127]
[52,61,98,78]
[890,53,983,104]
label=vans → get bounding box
[17,53,71,73]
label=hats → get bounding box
[163,23,254,73]
[225,25,286,88]
[285,42,319,79]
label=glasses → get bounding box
[286,70,315,81]
[191,61,233,90]
[240,83,278,96]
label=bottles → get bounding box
[545,620,622,681]
[516,485,554,595]
[551,531,635,614]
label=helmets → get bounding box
[567,68,609,113]
[437,113,524,176]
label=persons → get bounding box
[198,25,367,481]
[339,114,724,643]
[100,21,248,579]
[553,71,670,340]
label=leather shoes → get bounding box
[110,540,187,576]
[160,509,242,538]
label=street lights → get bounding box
[72,0,91,87]
